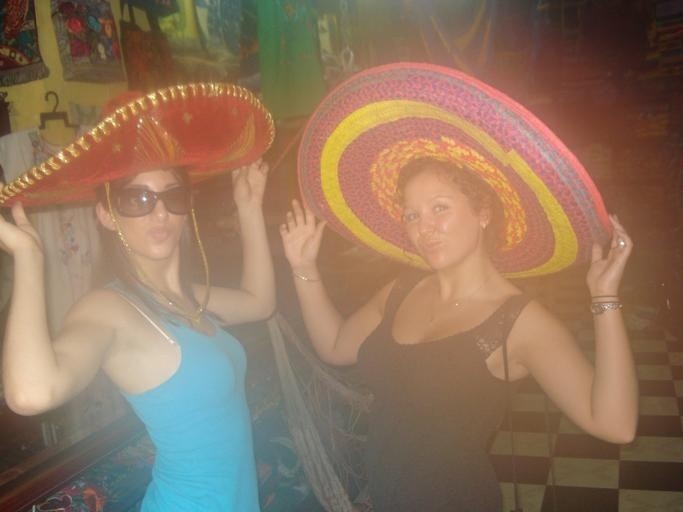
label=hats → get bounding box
[2,81,275,214]
[294,62,616,294]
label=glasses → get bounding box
[116,184,192,218]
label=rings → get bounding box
[617,236,625,250]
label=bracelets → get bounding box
[292,273,321,283]
[587,294,624,314]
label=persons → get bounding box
[276,157,639,512]
[0,160,272,512]
